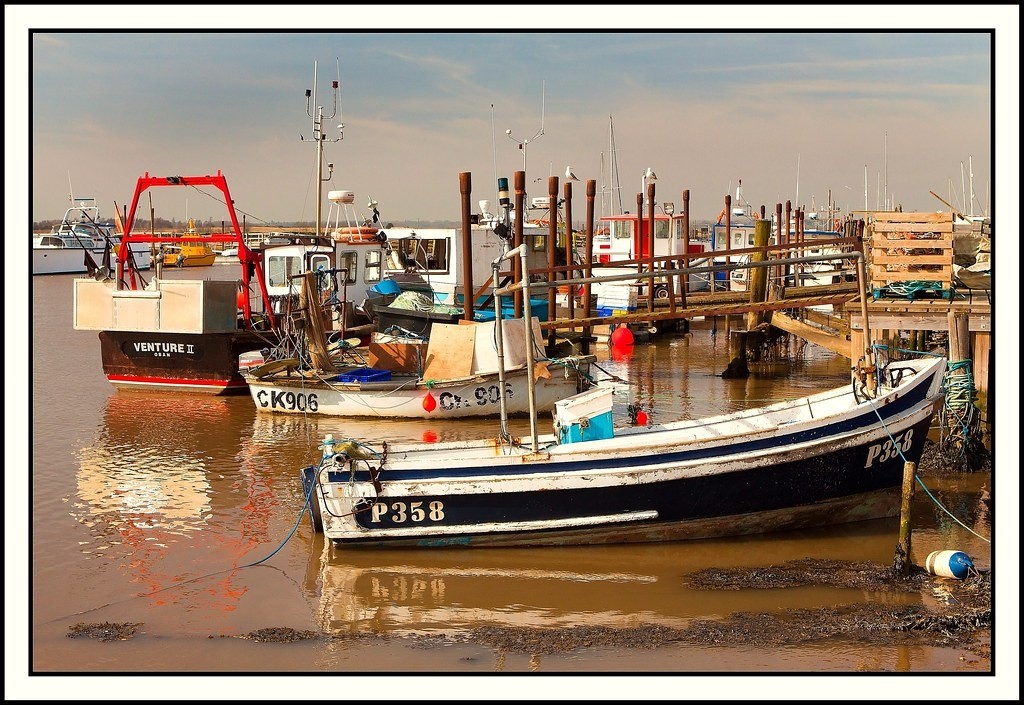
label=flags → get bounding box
[102,239,111,269]
[83,251,97,277]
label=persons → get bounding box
[899,204,902,213]
[895,207,899,213]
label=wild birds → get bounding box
[565,165,580,181]
[646,168,659,182]
[895,203,902,211]
[534,177,542,183]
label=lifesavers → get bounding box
[336,234,378,241]
[337,227,379,234]
[536,220,549,225]
[835,219,840,231]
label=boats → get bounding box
[236,263,602,422]
[297,239,953,552]
[32,194,152,276]
[71,57,991,397]
